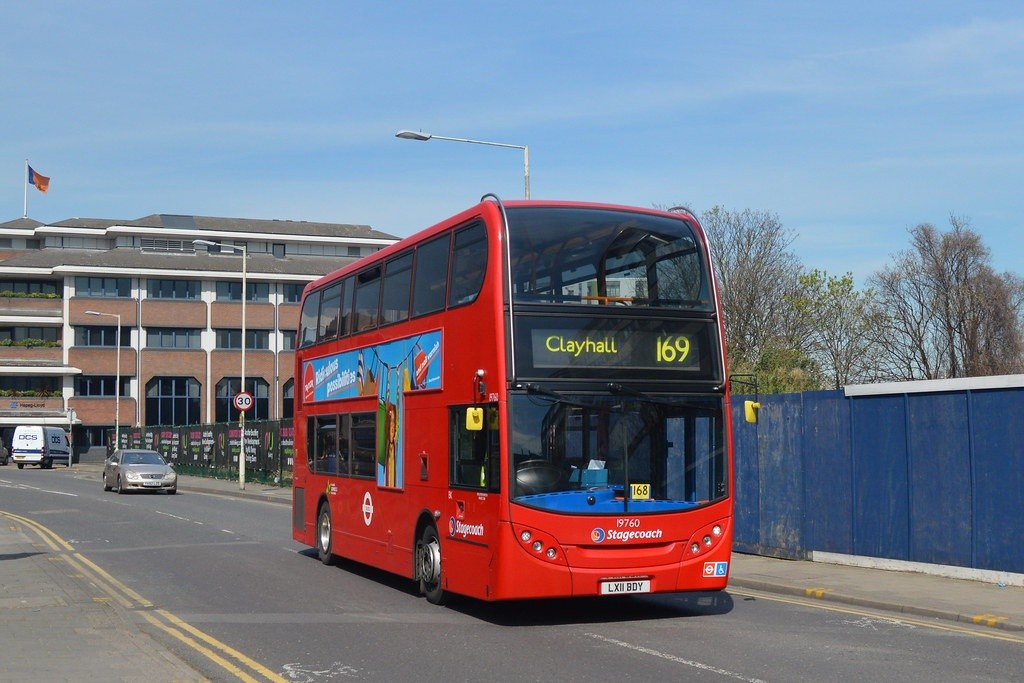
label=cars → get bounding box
[102,448,178,494]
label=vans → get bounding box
[12,425,72,468]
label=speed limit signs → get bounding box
[236,394,254,410]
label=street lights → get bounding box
[193,239,248,490]
[85,310,121,453]
[397,130,529,201]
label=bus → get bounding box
[292,194,760,606]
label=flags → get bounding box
[28,165,51,196]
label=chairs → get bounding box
[512,458,571,498]
[460,459,486,487]
[310,451,374,475]
